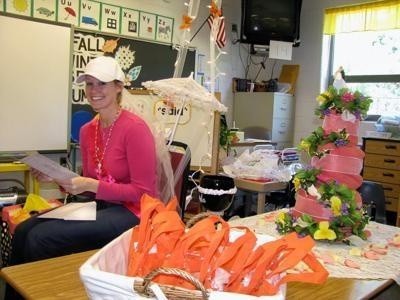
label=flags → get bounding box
[206,3,228,49]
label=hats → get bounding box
[75,55,126,84]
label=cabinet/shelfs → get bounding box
[359,136,400,228]
[231,91,295,153]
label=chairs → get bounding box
[159,139,191,220]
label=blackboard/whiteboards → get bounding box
[0,10,196,154]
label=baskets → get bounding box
[78,224,287,300]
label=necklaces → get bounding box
[93,104,123,181]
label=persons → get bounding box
[4,55,166,300]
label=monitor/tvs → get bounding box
[238,0,302,47]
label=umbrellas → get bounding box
[140,69,230,149]
[63,6,76,22]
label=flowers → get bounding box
[274,85,375,244]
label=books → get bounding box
[272,149,297,154]
[359,122,387,132]
[282,161,298,165]
[279,155,297,158]
[381,117,399,126]
[0,189,18,197]
[34,199,98,222]
[360,130,392,139]
[281,157,299,161]
[360,114,382,124]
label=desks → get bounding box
[1,205,400,300]
[218,174,289,216]
